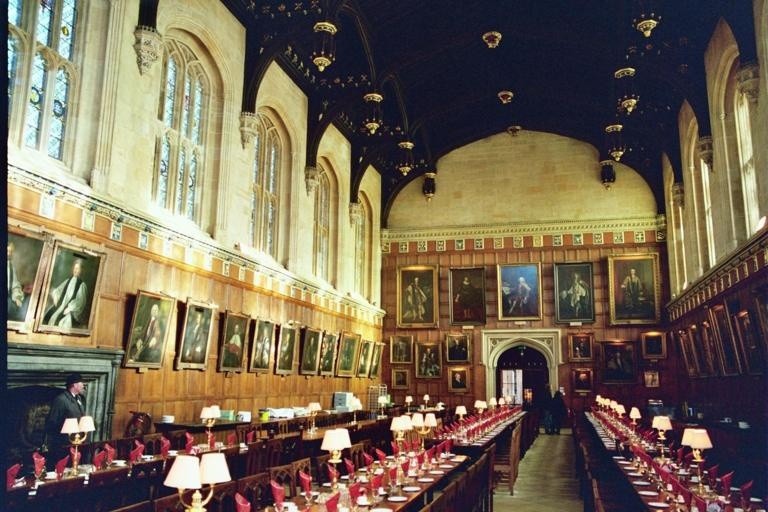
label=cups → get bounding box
[738,422,750,429]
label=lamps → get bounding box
[313,0,435,205]
[482,31,502,49]
[497,89,514,105]
[598,1,661,191]
[507,125,522,137]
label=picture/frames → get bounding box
[678,280,767,381]
[38,193,56,219]
[607,253,662,327]
[568,332,667,393]
[448,267,487,326]
[396,265,440,330]
[7,223,105,336]
[121,291,385,380]
[380,230,667,254]
[137,232,149,250]
[222,262,230,277]
[81,208,96,233]
[553,261,596,324]
[110,221,122,242]
[388,333,471,393]
[496,262,543,321]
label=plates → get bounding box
[268,447,467,512]
[609,452,767,512]
[159,406,310,450]
[432,410,528,446]
[584,408,672,453]
[12,439,249,497]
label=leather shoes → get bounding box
[545,430,560,436]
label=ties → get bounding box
[75,395,82,408]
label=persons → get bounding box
[544,385,566,435]
[45,373,89,472]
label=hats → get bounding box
[63,373,88,386]
